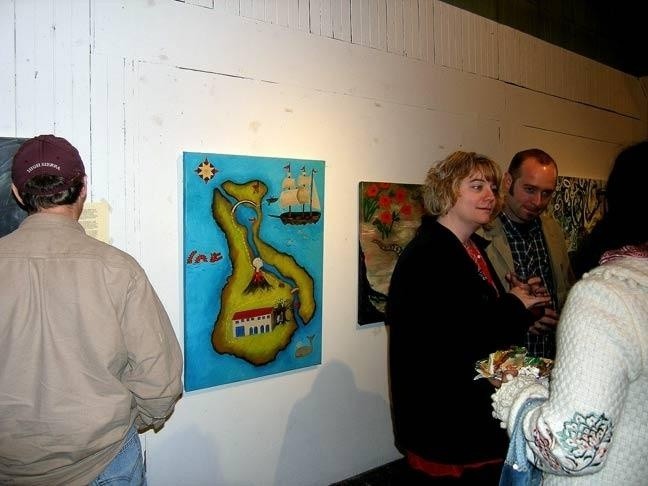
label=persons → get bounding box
[387,149,551,486]
[473,143,648,486]
[0,133,184,486]
[481,149,569,363]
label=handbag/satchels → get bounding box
[499,459,542,486]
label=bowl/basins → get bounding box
[475,354,554,387]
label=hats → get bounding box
[11,135,85,195]
